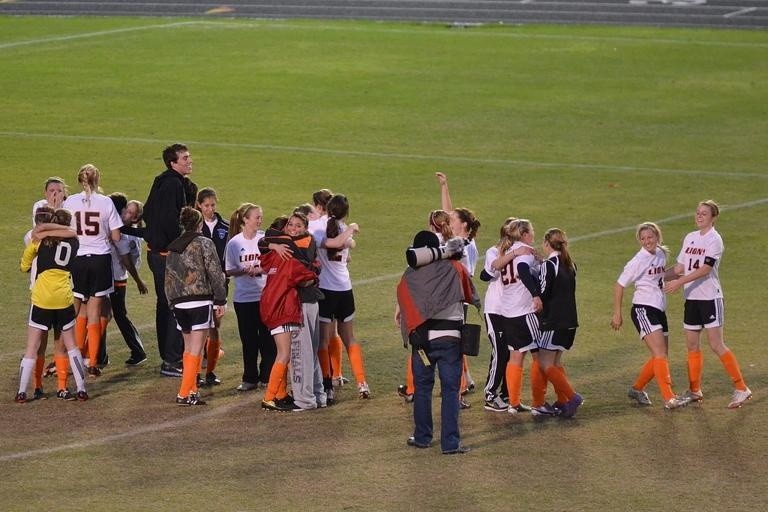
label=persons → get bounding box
[610,221,691,409]
[663,201,753,408]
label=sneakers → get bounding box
[331,377,344,387]
[398,385,414,403]
[629,387,703,408]
[407,436,431,448]
[236,383,259,391]
[442,446,470,453]
[15,366,101,401]
[460,384,583,417]
[261,395,303,412]
[125,355,147,366]
[358,383,370,399]
[728,387,752,408]
[161,361,221,405]
